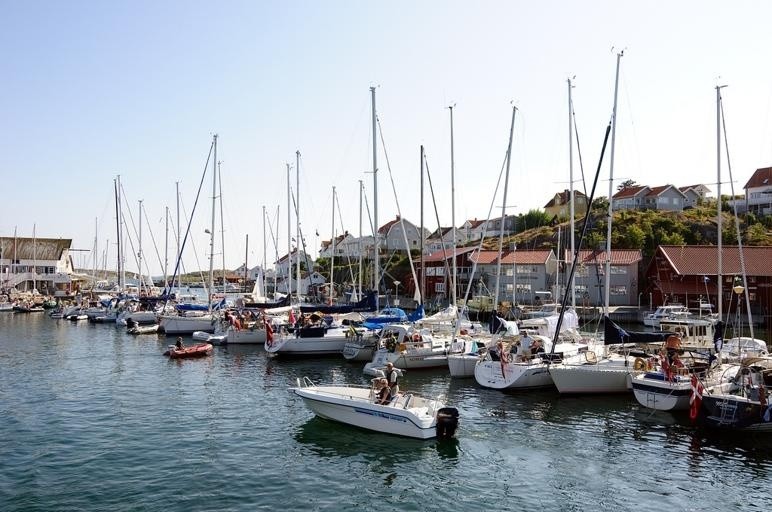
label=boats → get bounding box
[286,375,459,444]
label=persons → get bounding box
[666,331,681,365]
[176,336,184,350]
[379,379,391,405]
[386,362,399,396]
[515,332,539,361]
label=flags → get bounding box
[663,355,672,387]
[612,322,624,337]
[688,373,703,423]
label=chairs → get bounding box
[384,385,414,410]
[537,352,565,364]
